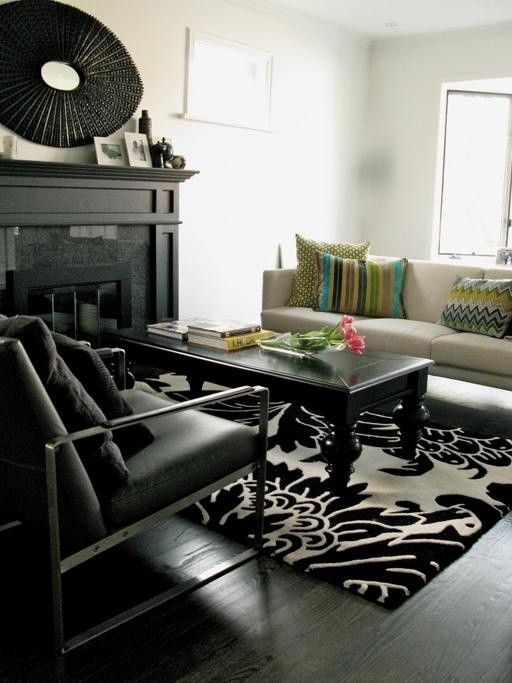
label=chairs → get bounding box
[0,309,272,683]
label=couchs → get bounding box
[260,255,512,393]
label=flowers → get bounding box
[260,313,367,359]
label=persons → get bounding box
[132,140,146,159]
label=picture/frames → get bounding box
[92,132,154,169]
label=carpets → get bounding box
[110,370,512,613]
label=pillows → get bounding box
[436,274,512,339]
[0,314,156,492]
[284,232,409,320]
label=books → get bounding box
[186,330,273,349]
[187,317,261,337]
[147,316,214,339]
